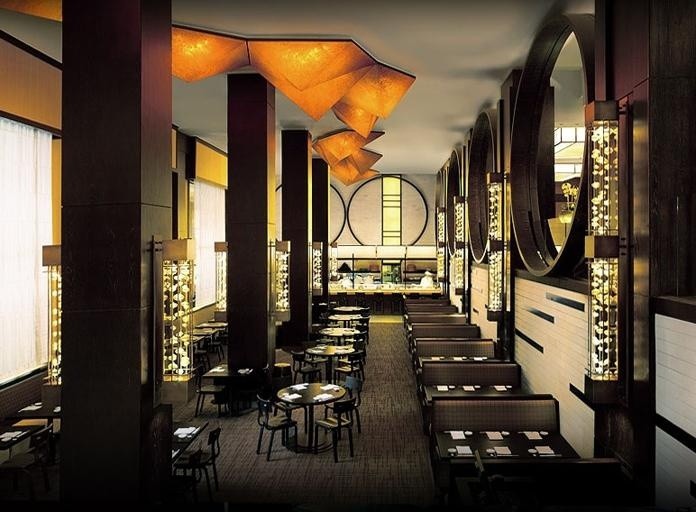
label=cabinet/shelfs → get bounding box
[402,270,438,283]
[342,271,381,282]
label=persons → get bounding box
[420,270,434,288]
[336,274,375,290]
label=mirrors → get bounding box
[509,10,595,279]
[466,107,498,268]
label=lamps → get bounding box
[310,130,389,186]
[435,205,450,285]
[451,193,469,295]
[150,230,202,409]
[484,170,509,322]
[308,240,327,294]
[212,240,227,323]
[267,237,294,324]
[170,22,419,139]
[40,241,62,408]
[583,97,624,404]
[559,181,580,223]
[328,240,339,283]
